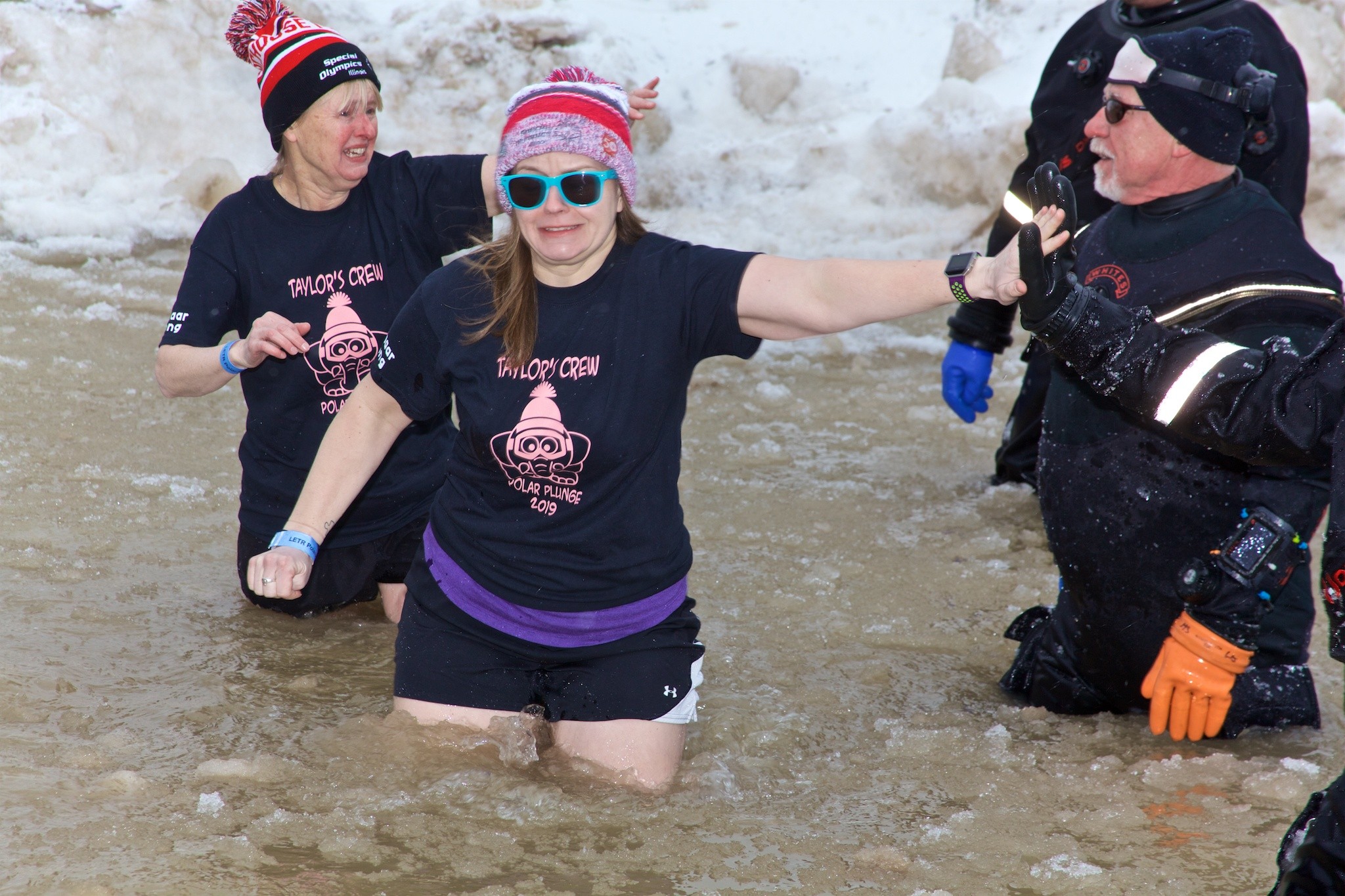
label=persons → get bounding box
[154,0,659,625]
[1002,28,1342,742]
[246,64,1069,789]
[941,0,1310,490]
[1018,161,1345,664]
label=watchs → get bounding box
[945,252,982,303]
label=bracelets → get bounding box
[268,530,319,561]
[220,339,245,374]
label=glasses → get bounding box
[1098,99,1145,124]
[500,169,618,210]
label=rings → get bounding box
[262,578,276,585]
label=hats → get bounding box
[495,68,636,214]
[227,0,380,152]
[1133,26,1256,165]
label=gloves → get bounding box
[1018,162,1079,321]
[1140,609,1256,741]
[941,341,993,424]
